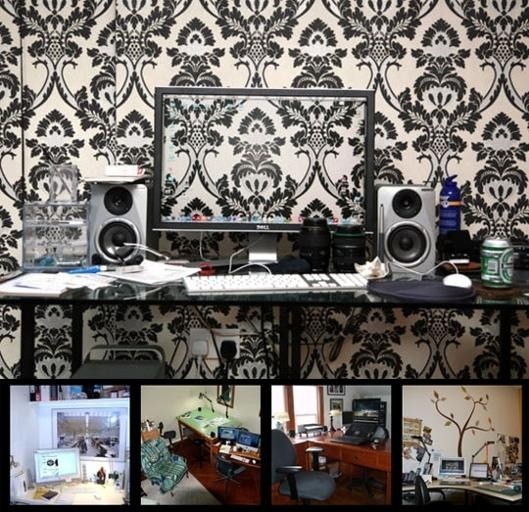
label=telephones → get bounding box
[403,470,415,483]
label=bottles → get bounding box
[144,419,153,432]
[96,466,106,484]
[298,219,331,268]
[116,479,120,490]
[439,182,461,237]
[333,225,366,269]
[492,456,501,469]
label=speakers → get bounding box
[86,180,157,263]
[374,182,436,280]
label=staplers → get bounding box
[197,261,215,276]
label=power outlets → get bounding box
[189,326,241,360]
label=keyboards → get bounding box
[185,273,369,294]
[229,454,249,463]
[478,483,510,492]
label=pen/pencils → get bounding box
[68,265,116,273]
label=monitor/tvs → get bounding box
[439,458,466,479]
[33,447,80,484]
[218,427,260,453]
[152,85,376,271]
[469,463,491,482]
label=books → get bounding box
[1,272,75,299]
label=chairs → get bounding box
[271,429,343,503]
[414,476,446,506]
[141,422,189,497]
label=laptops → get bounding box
[330,422,377,445]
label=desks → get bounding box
[402,469,524,503]
[309,431,391,503]
[1,255,527,382]
[177,406,261,472]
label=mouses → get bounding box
[252,459,257,464]
[442,275,473,290]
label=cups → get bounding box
[288,429,295,437]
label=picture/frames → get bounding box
[216,385,235,408]
[51,406,128,464]
[326,384,345,412]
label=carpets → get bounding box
[141,467,220,506]
